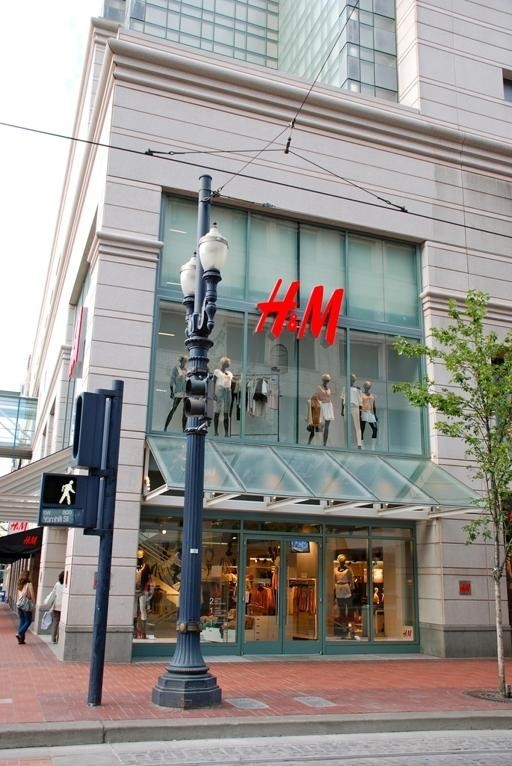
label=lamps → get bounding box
[373,557,379,567]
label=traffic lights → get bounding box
[41,472,76,506]
[68,391,105,470]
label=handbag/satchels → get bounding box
[16,595,35,612]
[41,612,52,630]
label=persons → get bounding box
[305,374,335,447]
[211,355,234,437]
[164,354,189,433]
[340,373,363,451]
[45,570,65,644]
[14,570,36,644]
[334,554,356,640]
[359,380,378,451]
[133,550,151,638]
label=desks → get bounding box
[361,604,384,637]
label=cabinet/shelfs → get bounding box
[201,566,278,642]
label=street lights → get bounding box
[149,176,232,712]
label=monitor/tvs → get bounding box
[290,540,310,553]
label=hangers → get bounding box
[246,372,272,381]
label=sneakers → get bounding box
[16,634,25,644]
